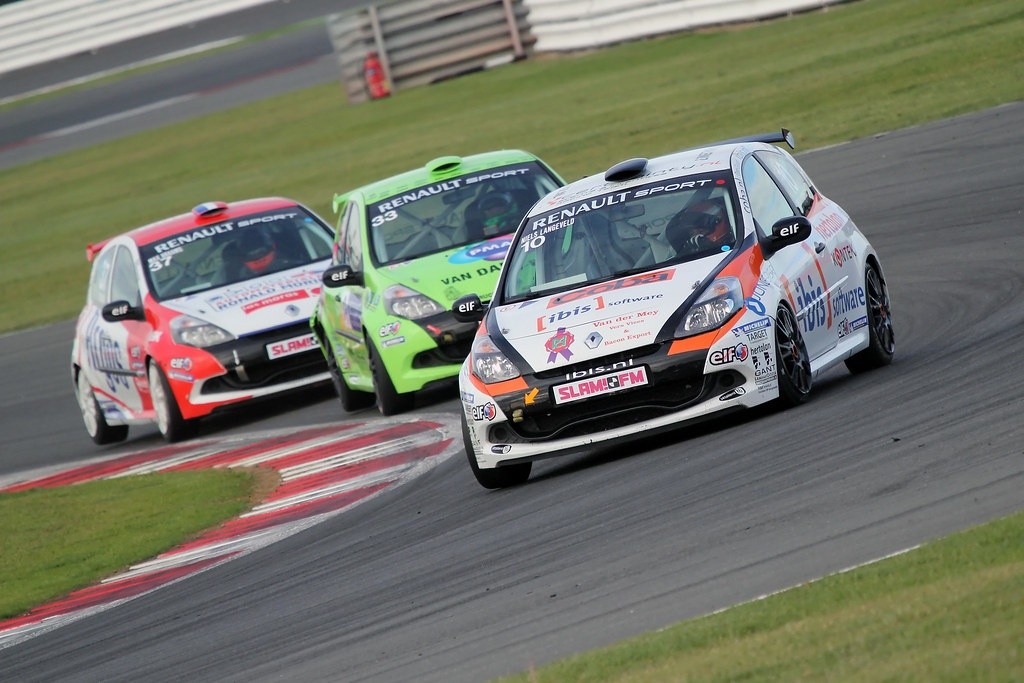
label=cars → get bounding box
[62,195,352,445]
[446,129,896,494]
[304,147,583,420]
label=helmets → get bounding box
[236,225,273,259]
[478,191,520,227]
[685,200,730,241]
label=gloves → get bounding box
[685,233,707,250]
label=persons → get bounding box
[475,190,524,237]
[670,200,733,256]
[230,225,303,279]
